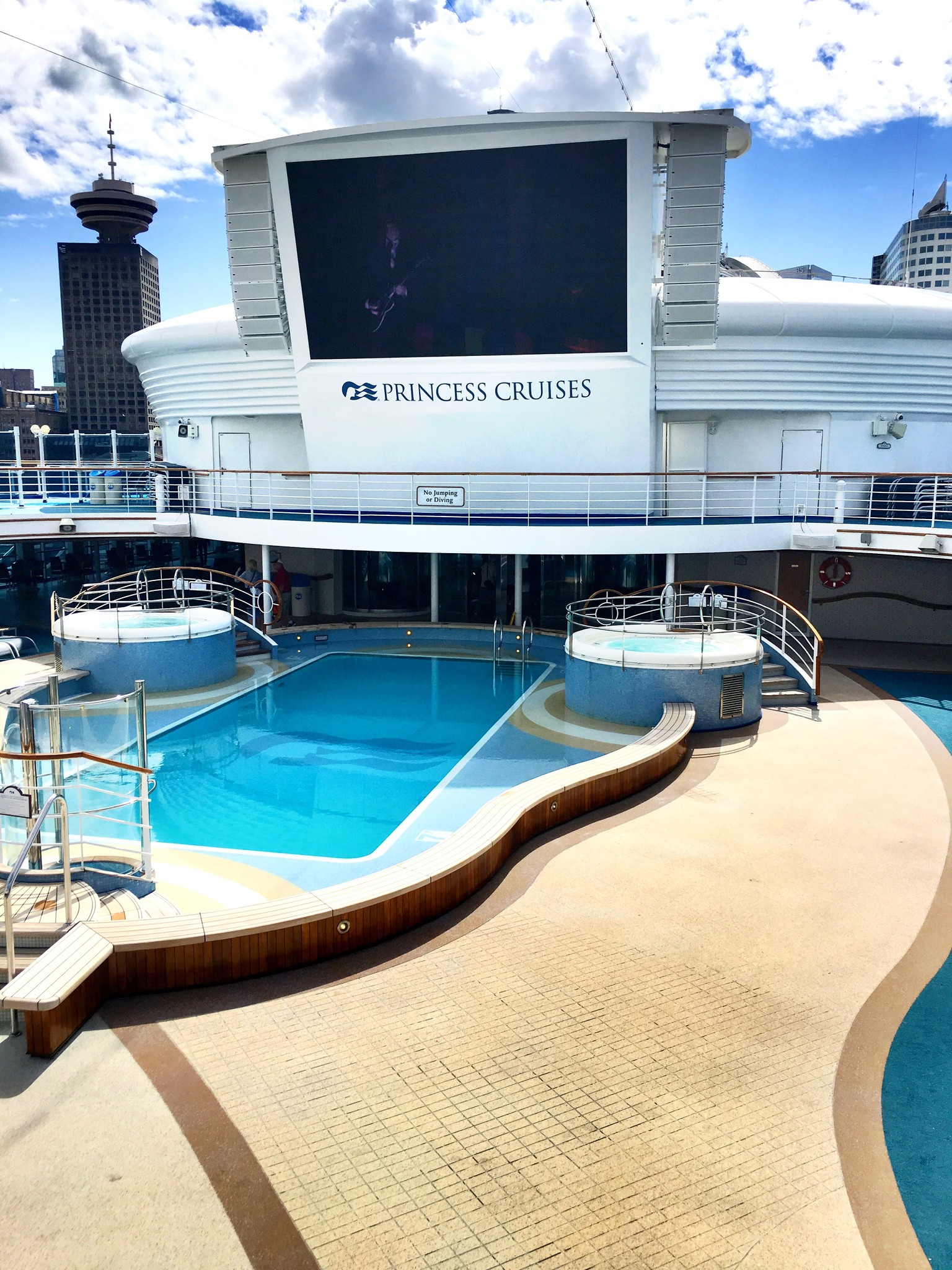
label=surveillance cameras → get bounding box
[897,414,903,421]
[178,419,184,424]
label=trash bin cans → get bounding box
[104,470,122,505]
[705,585,752,633]
[89,470,106,504]
[291,577,311,623]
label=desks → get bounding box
[0,627,17,636]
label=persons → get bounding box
[235,559,263,632]
[363,224,419,357]
[270,558,296,629]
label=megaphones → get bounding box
[59,517,77,534]
[919,534,940,554]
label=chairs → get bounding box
[864,478,952,522]
[0,633,39,659]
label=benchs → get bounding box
[3,668,89,707]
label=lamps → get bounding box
[178,424,198,437]
[918,534,944,554]
[872,420,907,440]
[59,517,77,536]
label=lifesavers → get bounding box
[820,558,850,589]
